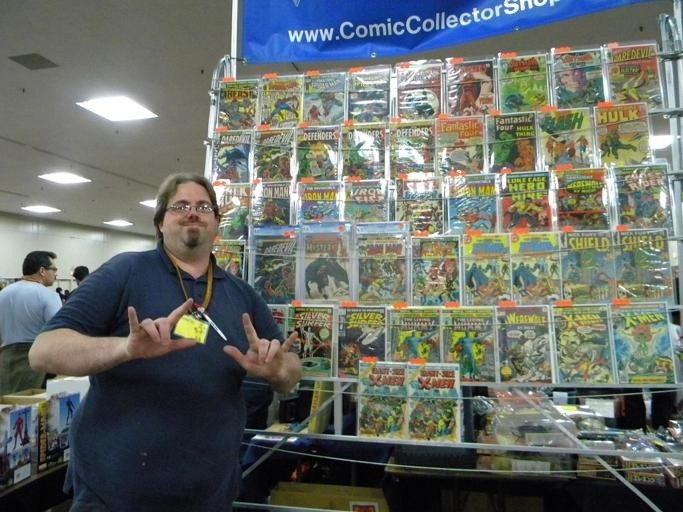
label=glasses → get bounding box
[163,203,218,216]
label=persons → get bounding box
[28,170,303,512]
[66,397,75,423]
[0,250,63,394]
[13,412,23,450]
[453,327,489,381]
[302,326,321,359]
[402,331,438,361]
[74,266,89,284]
[507,198,544,228]
[264,95,298,124]
